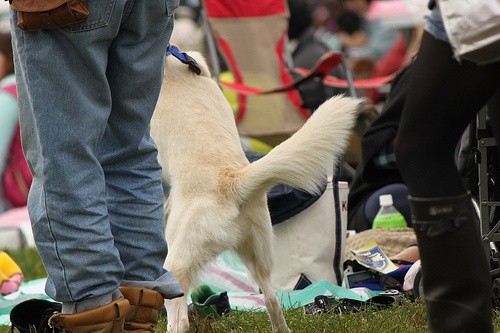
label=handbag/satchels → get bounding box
[247,180,350,295]
[241,153,327,225]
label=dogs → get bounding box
[149,18,367,333]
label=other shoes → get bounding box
[118,286,163,333]
[188,285,231,323]
[49,298,129,333]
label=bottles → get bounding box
[372,194,408,229]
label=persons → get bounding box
[285,0,500,333]
[7,0,182,333]
[0,33,34,216]
